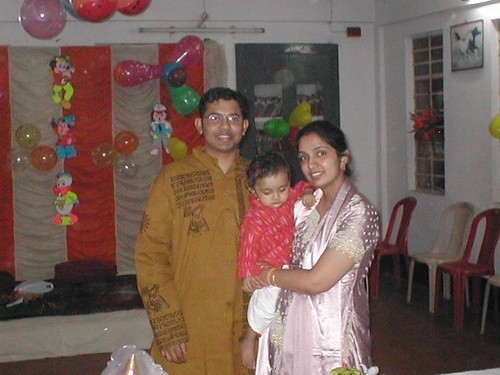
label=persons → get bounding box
[254,119,382,375]
[238,151,318,370]
[135,87,259,375]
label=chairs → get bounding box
[434,208,500,333]
[369,196,417,299]
[479,273,500,335]
[406,201,477,313]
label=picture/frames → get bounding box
[450,19,485,73]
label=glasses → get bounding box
[204,114,243,123]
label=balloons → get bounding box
[166,137,188,158]
[20,0,67,41]
[114,0,151,16]
[29,146,58,170]
[288,103,313,127]
[263,120,290,138]
[16,124,40,149]
[488,114,500,139]
[115,131,139,155]
[61,0,82,19]
[113,156,140,179]
[70,0,117,23]
[92,143,122,165]
[4,146,31,170]
[290,125,302,146]
[113,35,203,116]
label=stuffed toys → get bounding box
[52,172,79,225]
[50,114,78,159]
[150,104,173,163]
[50,55,76,109]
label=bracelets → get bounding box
[266,267,279,287]
